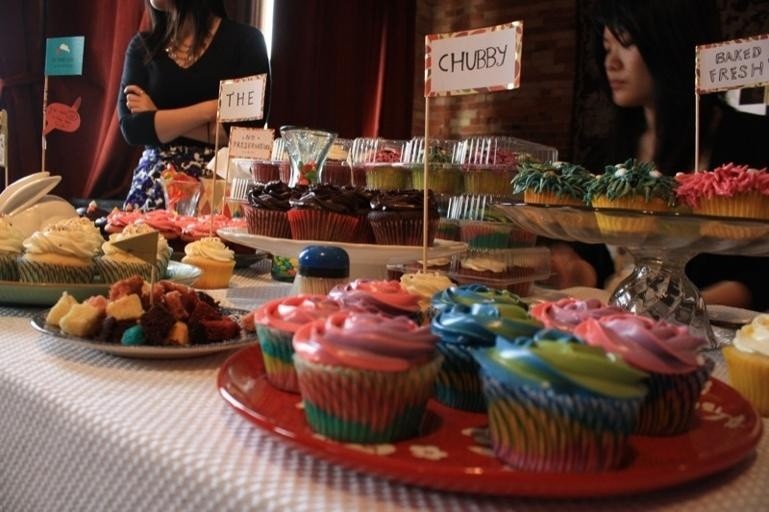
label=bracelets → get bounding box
[205,123,212,146]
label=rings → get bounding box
[139,90,145,95]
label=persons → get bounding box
[533,2,769,315]
[115,1,271,218]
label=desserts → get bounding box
[0,141,769,474]
